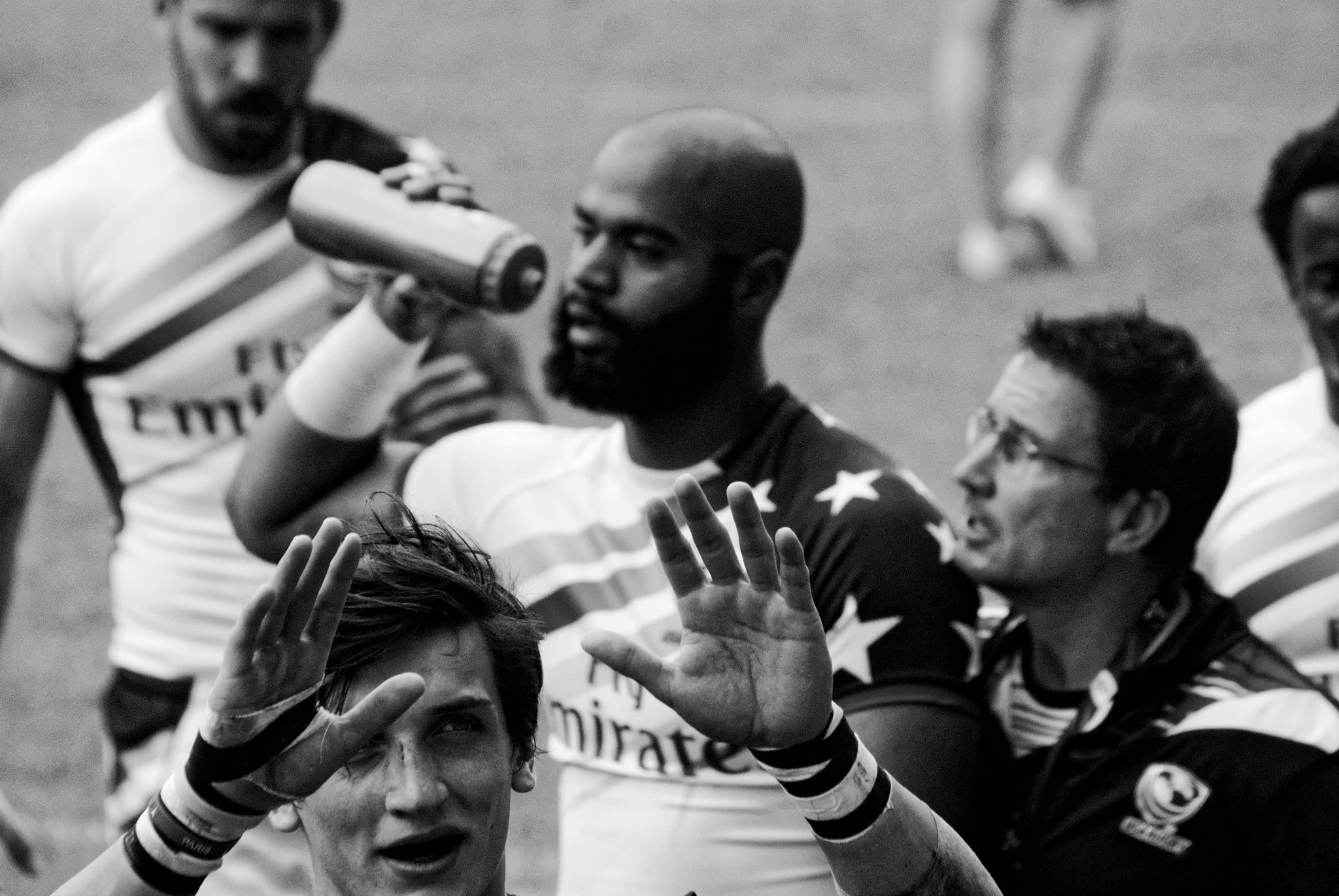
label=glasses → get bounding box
[965,409,1097,477]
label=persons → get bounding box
[52,471,1002,896]
[0,0,538,842]
[932,0,1126,284]
[951,291,1339,896]
[1194,106,1339,709]
[224,106,983,896]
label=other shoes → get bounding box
[959,222,1046,279]
[1002,156,1108,277]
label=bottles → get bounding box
[289,158,546,314]
